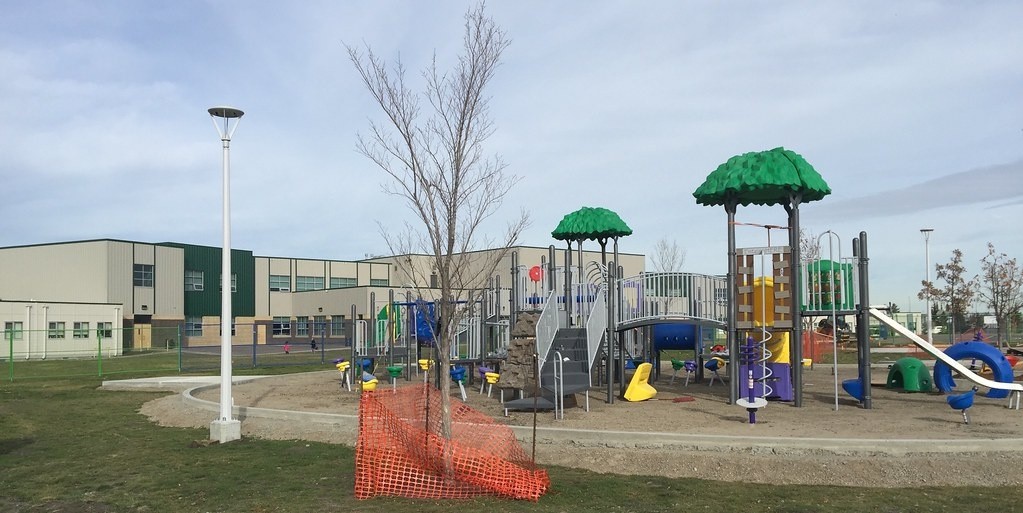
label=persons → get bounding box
[283,341,289,354]
[310,338,316,350]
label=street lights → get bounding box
[920,229,935,346]
[207,107,244,422]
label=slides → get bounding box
[870,308,1023,391]
